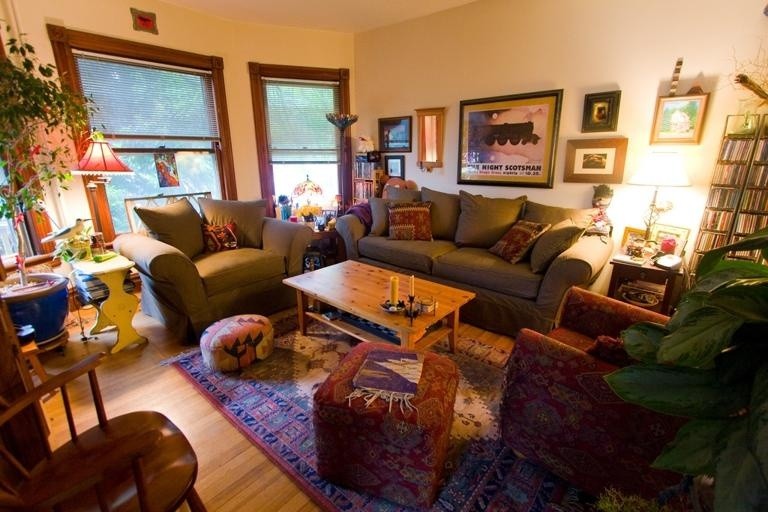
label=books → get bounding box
[620,278,666,302]
[352,161,383,201]
[686,138,768,290]
[71,269,136,304]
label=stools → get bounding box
[313,342,459,510]
[200,314,274,371]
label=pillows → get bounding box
[134,197,267,258]
[368,185,601,274]
[585,332,642,367]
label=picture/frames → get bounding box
[378,116,412,179]
[619,223,691,257]
[457,89,709,188]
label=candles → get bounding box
[390,275,414,306]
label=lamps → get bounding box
[71,141,134,249]
[627,147,692,243]
[291,113,358,215]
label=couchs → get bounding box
[335,201,615,339]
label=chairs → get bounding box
[500,285,704,512]
[112,217,315,344]
[0,357,208,512]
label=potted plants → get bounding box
[0,18,106,361]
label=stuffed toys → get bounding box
[584,182,614,236]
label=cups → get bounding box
[289,216,296,222]
[318,223,325,231]
[90,232,106,251]
[327,221,336,230]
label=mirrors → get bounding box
[413,107,446,173]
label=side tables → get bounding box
[607,260,684,315]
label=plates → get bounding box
[377,302,407,314]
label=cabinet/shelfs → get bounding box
[687,114,768,290]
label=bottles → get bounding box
[90,236,104,258]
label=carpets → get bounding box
[171,314,602,512]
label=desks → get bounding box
[71,249,148,354]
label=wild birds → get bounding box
[40,217,94,244]
